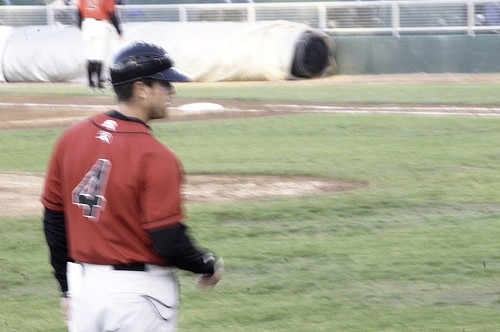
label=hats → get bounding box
[110,41,193,84]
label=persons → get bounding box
[40,41,224,331]
[75,0,122,89]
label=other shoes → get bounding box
[89,80,106,88]
